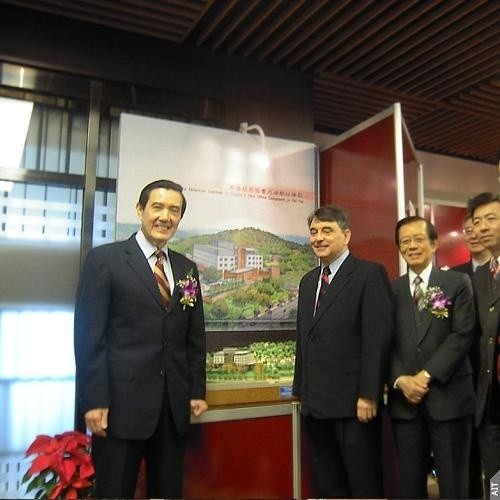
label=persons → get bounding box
[384,214,478,500]
[466,191,499,499]
[287,204,396,500]
[446,211,493,500]
[72,179,209,500]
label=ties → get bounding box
[412,275,425,313]
[489,259,499,279]
[152,250,172,312]
[315,265,332,318]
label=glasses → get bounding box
[462,229,473,235]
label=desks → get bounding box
[182,399,313,499]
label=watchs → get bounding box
[422,369,435,382]
